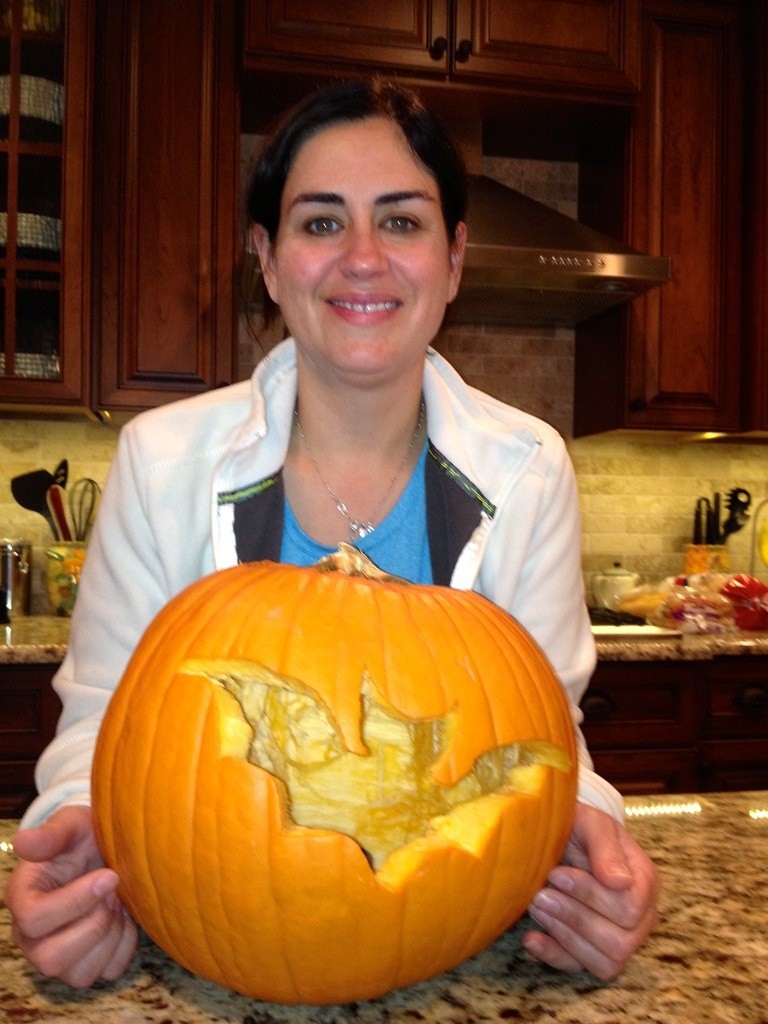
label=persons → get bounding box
[5,80,660,990]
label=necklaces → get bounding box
[294,395,426,543]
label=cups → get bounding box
[0,538,33,617]
[683,544,732,574]
[47,541,89,617]
[593,562,640,611]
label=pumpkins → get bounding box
[89,542,579,1005]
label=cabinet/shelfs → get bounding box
[579,654,703,796]
[572,0,767,445]
[90,0,242,410]
[243,0,645,98]
[704,654,767,791]
[1,661,67,820]
[0,0,104,420]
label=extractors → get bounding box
[242,173,672,325]
